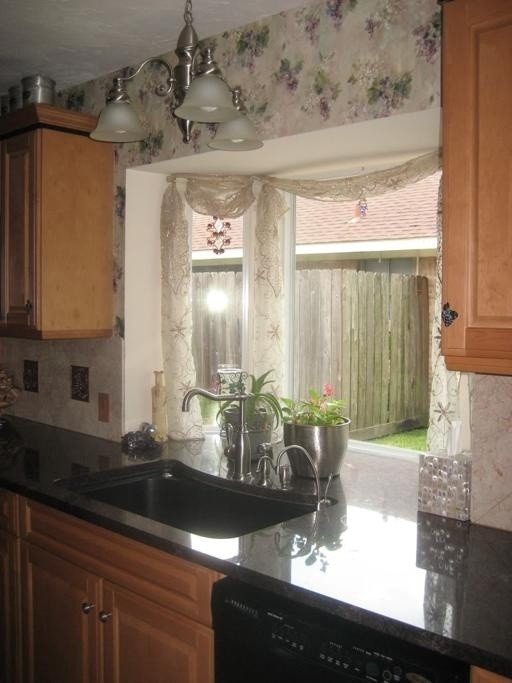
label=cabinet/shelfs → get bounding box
[1,102,116,342]
[17,500,216,682]
[440,0,511,377]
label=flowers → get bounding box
[280,382,351,425]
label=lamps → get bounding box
[85,0,265,152]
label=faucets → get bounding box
[256,456,289,491]
[180,387,254,481]
[275,444,321,512]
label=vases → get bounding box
[281,415,353,481]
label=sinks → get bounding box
[58,460,337,540]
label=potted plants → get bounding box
[213,367,284,465]
